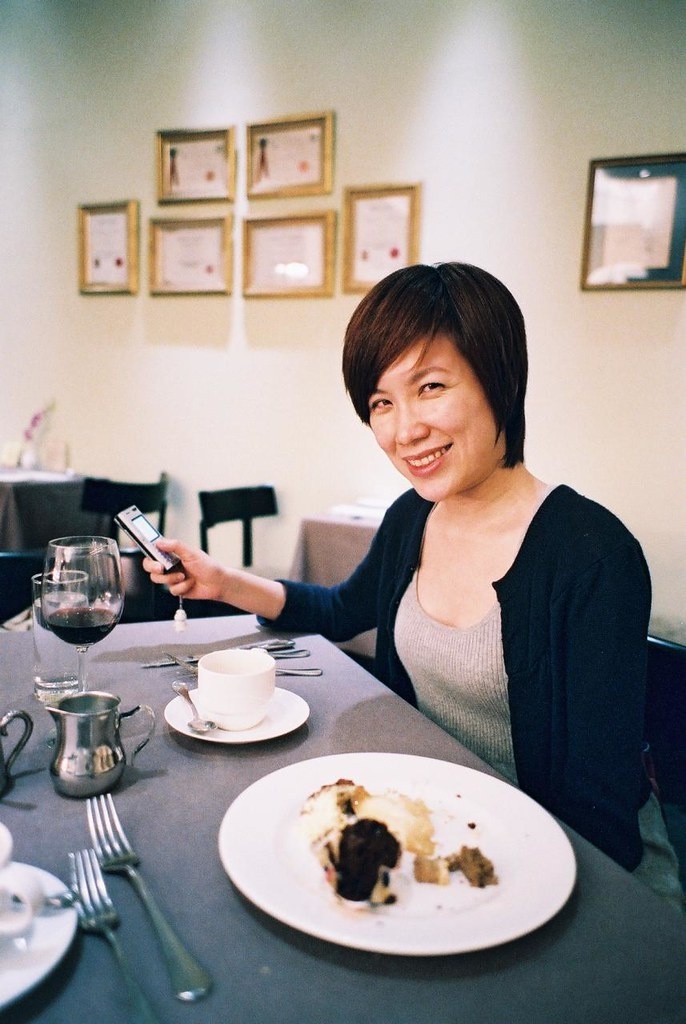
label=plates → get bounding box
[218,753,576,955]
[0,862,77,1010]
[163,687,310,744]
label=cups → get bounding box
[31,571,88,702]
[197,649,276,730]
[1,822,43,944]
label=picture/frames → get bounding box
[243,211,335,299]
[246,110,333,200]
[77,199,139,296]
[578,151,686,290]
[148,214,233,296]
[154,125,236,206]
[340,182,421,295]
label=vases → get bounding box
[18,443,39,465]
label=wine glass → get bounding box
[41,535,123,692]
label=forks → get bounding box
[160,649,323,678]
[85,793,213,1004]
[67,849,162,1023]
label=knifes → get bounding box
[140,638,295,668]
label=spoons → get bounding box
[171,680,219,733]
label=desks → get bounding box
[289,494,391,659]
[0,614,686,1024]
[0,466,111,553]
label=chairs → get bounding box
[198,485,290,581]
[0,549,162,628]
[644,635,686,811]
[78,472,168,545]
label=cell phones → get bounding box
[112,505,182,572]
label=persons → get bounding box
[142,261,686,934]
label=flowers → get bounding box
[17,401,57,442]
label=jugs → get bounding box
[0,709,34,800]
[42,691,155,798]
